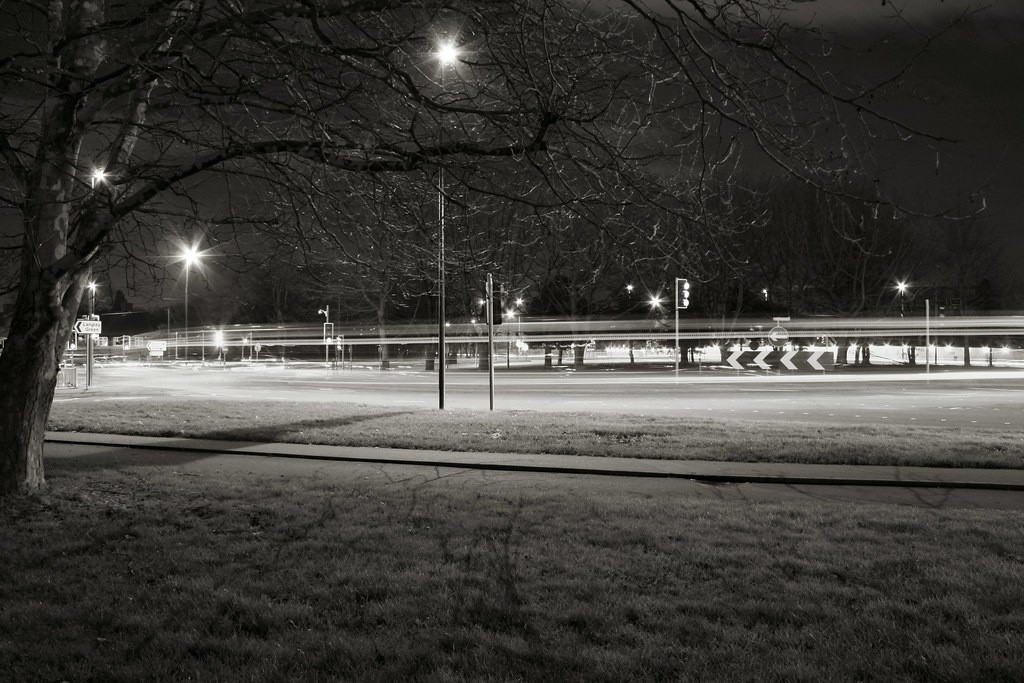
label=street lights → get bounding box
[318,304,329,365]
[897,282,906,359]
[184,249,193,359]
[438,39,457,411]
[507,311,513,369]
[87,281,96,386]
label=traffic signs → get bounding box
[74,321,102,334]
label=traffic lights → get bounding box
[676,279,687,309]
[337,335,344,351]
[324,323,334,344]
